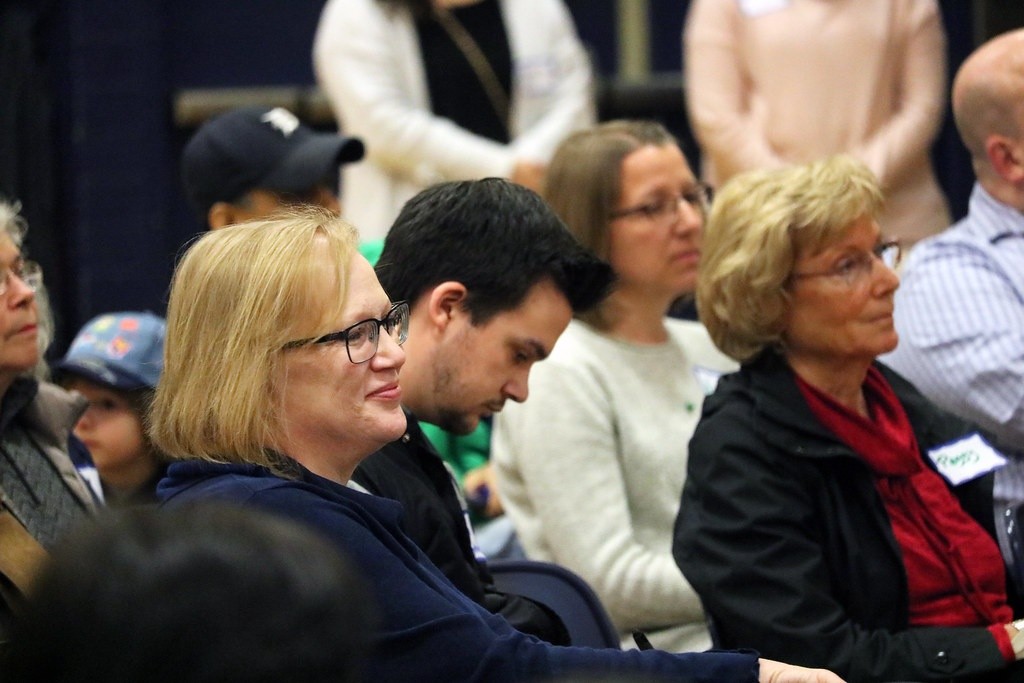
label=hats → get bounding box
[180,103,363,203]
[59,311,169,393]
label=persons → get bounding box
[1,30,1024,683]
[680,0,951,255]
[312,0,597,246]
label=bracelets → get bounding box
[1012,618,1024,631]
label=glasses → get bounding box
[603,181,715,229]
[789,241,902,285]
[280,301,409,364]
[0,260,43,296]
